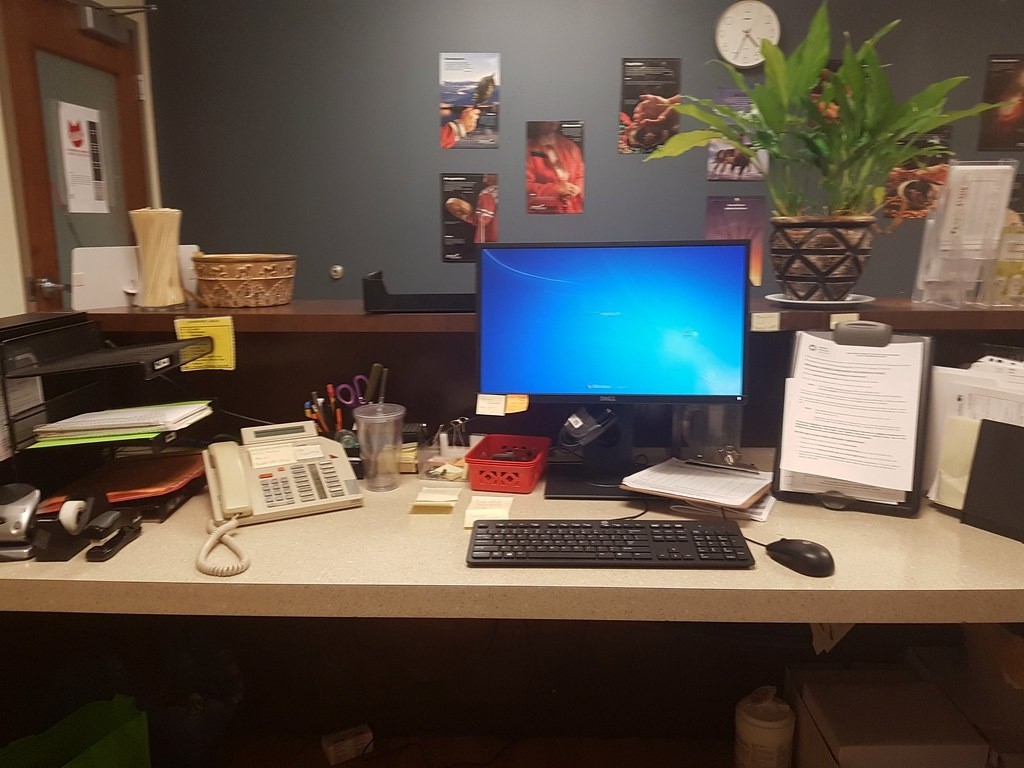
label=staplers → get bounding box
[84,506,143,562]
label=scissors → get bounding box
[335,375,369,407]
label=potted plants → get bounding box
[642,0,1022,302]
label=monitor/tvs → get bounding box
[476,239,749,497]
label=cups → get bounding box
[683,403,743,462]
[351,403,407,492]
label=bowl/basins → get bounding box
[190,253,298,308]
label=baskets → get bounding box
[465,432,551,493]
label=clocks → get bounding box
[711,0,782,70]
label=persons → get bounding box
[441,105,480,150]
[524,120,584,215]
[634,93,682,130]
[448,175,498,244]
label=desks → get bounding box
[1,303,1024,768]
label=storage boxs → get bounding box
[785,667,992,768]
[465,434,551,493]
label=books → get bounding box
[33,403,214,440]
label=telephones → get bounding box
[201,419,365,528]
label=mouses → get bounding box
[766,538,835,577]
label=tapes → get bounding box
[58,500,87,535]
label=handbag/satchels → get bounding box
[0,690,149,768]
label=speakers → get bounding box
[0,483,43,561]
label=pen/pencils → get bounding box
[304,383,342,432]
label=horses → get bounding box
[711,144,754,177]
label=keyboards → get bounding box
[466,520,756,570]
